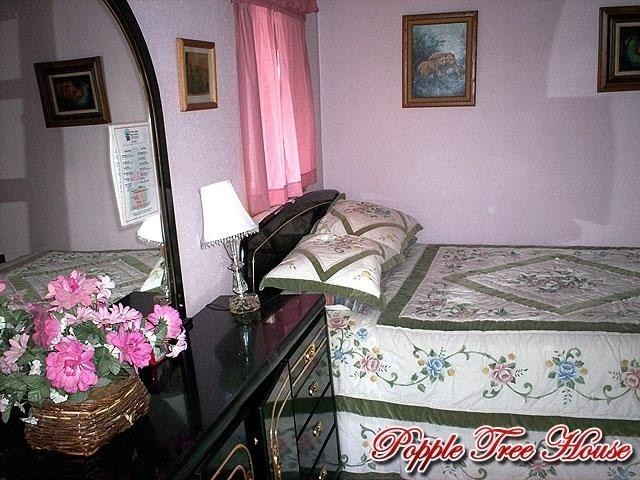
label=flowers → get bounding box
[0,270,188,425]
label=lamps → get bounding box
[136,188,173,307]
[198,179,261,314]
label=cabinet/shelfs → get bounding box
[107,291,168,318]
[0,294,342,480]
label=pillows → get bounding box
[259,198,424,317]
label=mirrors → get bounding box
[0,0,193,334]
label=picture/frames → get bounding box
[597,5,640,93]
[33,55,112,128]
[176,37,218,112]
[401,10,478,108]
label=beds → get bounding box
[239,190,640,480]
[0,249,167,314]
